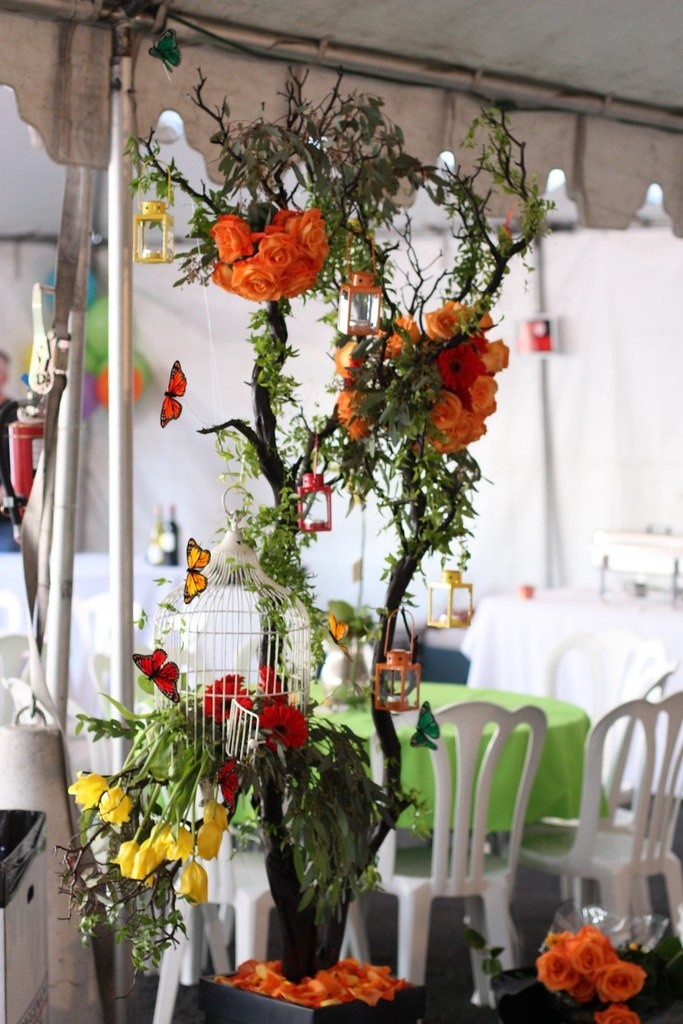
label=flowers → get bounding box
[212,198,327,302]
[536,926,647,1024]
[67,665,310,907]
[333,301,510,455]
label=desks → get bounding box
[457,583,683,802]
[191,681,606,1024]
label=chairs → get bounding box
[459,628,669,951]
[501,659,683,945]
[339,701,547,1010]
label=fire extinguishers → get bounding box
[3,413,47,546]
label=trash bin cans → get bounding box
[0,809,49,1024]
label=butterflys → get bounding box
[327,613,356,664]
[182,538,211,604]
[160,360,187,429]
[132,649,181,703]
[409,701,440,751]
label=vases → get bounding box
[196,959,426,1024]
